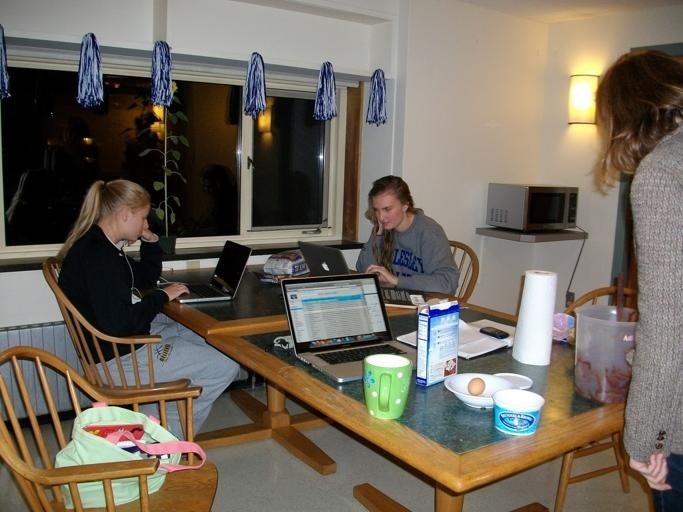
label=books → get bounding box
[395,319,515,360]
[379,286,427,309]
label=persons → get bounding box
[53,178,240,447]
[196,164,238,234]
[354,174,460,296]
[584,48,682,510]
[2,168,61,244]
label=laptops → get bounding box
[297,239,364,276]
[280,272,417,384]
[157,240,253,305]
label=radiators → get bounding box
[0,320,91,420]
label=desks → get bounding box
[203,305,628,512]
[149,262,463,476]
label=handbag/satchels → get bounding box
[54,399,207,512]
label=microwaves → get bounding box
[485,183,577,230]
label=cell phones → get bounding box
[481,326,509,339]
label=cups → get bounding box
[492,392,546,436]
[364,353,414,424]
[572,305,638,403]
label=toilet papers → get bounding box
[511,269,558,367]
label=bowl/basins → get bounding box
[445,372,510,408]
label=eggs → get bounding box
[469,377,485,395]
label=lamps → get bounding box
[564,73,596,125]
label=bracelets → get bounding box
[146,234,155,240]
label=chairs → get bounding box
[553,278,635,511]
[41,256,189,435]
[445,237,478,303]
[0,344,219,510]
